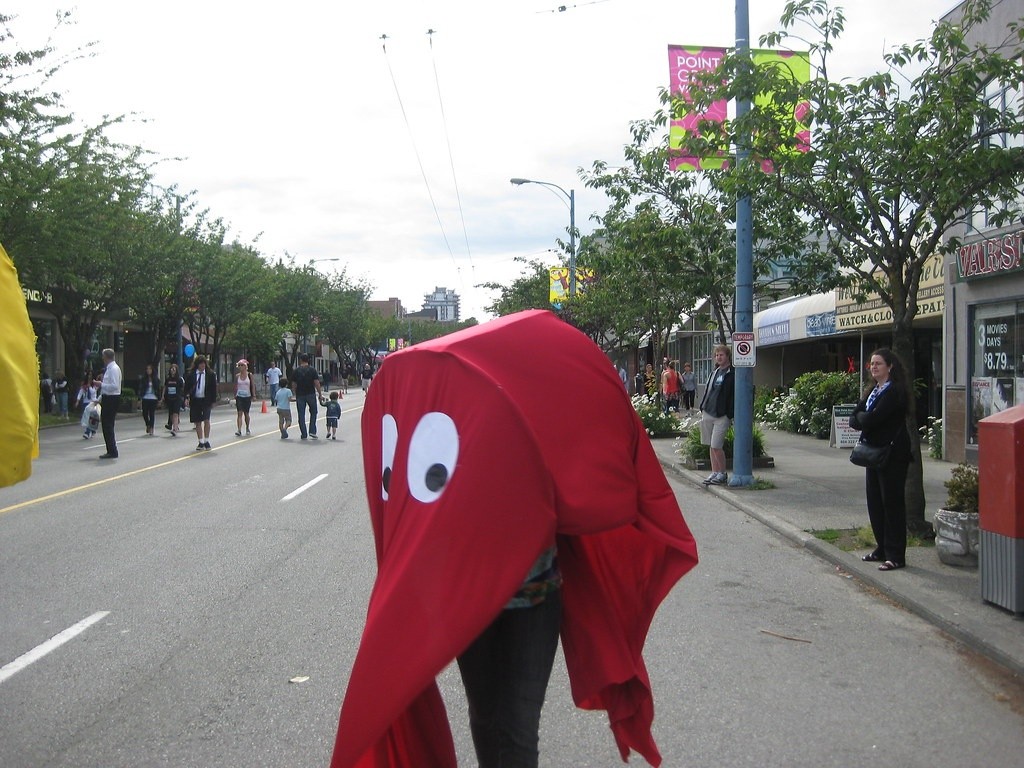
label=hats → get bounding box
[240,359,249,368]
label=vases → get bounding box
[687,455,775,470]
[648,431,689,439]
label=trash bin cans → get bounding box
[976,405,1024,615]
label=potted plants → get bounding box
[933,460,979,567]
[119,388,137,413]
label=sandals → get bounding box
[862,552,884,561]
[877,561,903,570]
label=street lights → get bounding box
[303,258,340,352]
[510,178,576,319]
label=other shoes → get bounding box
[246,429,250,434]
[165,425,172,430]
[196,443,203,450]
[281,429,288,438]
[83,434,89,439]
[146,424,153,433]
[205,442,211,450]
[310,433,318,438]
[92,433,96,437]
[302,436,307,439]
[326,434,330,438]
[332,437,336,439]
[100,453,114,457]
[235,431,240,436]
[171,430,176,436]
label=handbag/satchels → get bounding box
[81,402,102,430]
[49,394,57,406]
[676,372,686,395]
[850,438,895,474]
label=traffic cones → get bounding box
[261,400,269,413]
[338,390,344,399]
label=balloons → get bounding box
[184,344,195,358]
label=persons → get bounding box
[341,371,347,395]
[75,367,106,439]
[138,364,162,435]
[274,379,296,438]
[360,363,373,397]
[318,370,330,392]
[40,370,69,420]
[698,345,734,486]
[329,311,700,767]
[291,353,324,440]
[643,356,698,417]
[233,359,256,436]
[847,346,914,571]
[613,365,626,390]
[162,356,220,450]
[92,348,122,459]
[266,362,282,406]
[319,392,341,439]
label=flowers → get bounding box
[632,392,682,435]
[674,414,762,456]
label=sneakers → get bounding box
[703,473,717,485]
[711,472,728,484]
